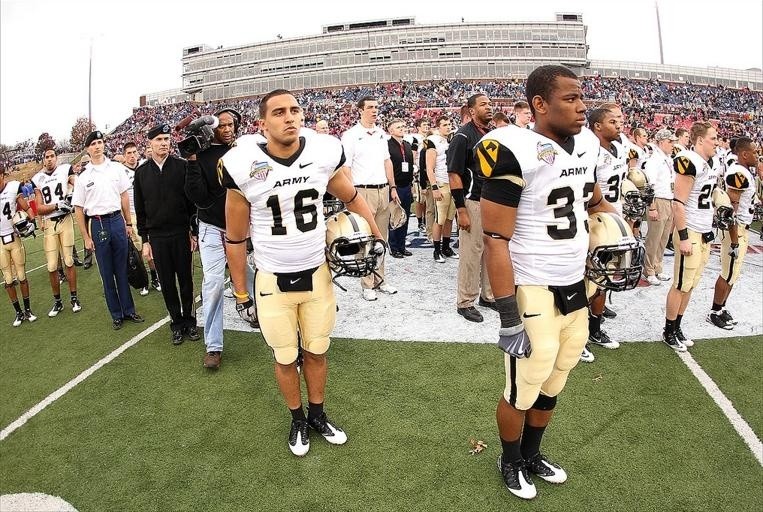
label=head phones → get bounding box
[214,108,241,133]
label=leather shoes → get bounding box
[204,351,223,370]
[456,295,500,324]
[110,312,146,331]
[387,225,460,264]
[251,320,260,329]
[169,324,201,345]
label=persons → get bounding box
[1,68,763,369]
[470,64,632,507]
[215,88,388,458]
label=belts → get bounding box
[88,210,120,219]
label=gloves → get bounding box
[727,246,740,258]
[496,324,533,359]
[232,290,259,323]
[369,240,389,271]
[56,193,76,213]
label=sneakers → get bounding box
[305,408,351,446]
[11,256,93,328]
[138,278,162,297]
[287,419,311,455]
[659,307,739,352]
[496,454,537,501]
[522,456,569,484]
[577,305,621,364]
[359,281,399,302]
[639,268,672,287]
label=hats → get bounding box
[84,130,103,145]
[654,128,679,142]
[386,199,407,231]
[147,124,171,139]
[211,106,240,129]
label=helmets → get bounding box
[11,210,36,237]
[711,187,734,231]
[627,167,655,204]
[580,211,642,290]
[325,213,386,278]
[620,178,646,222]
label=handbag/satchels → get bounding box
[126,236,149,289]
